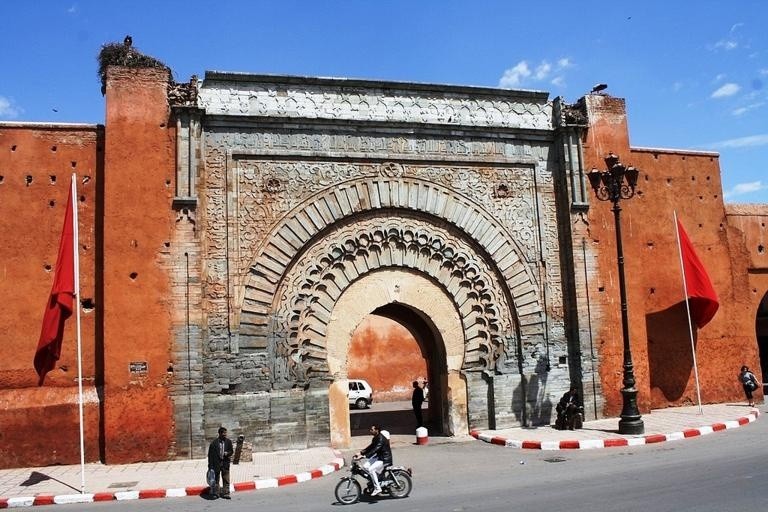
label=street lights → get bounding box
[585,149,645,434]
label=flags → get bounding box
[32,173,81,386]
[671,211,720,330]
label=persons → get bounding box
[352,423,393,497]
[207,426,233,500]
[739,366,761,407]
[555,385,584,430]
[411,381,423,429]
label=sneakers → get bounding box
[370,488,382,496]
[223,494,231,499]
[212,494,220,500]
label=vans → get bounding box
[346,379,373,410]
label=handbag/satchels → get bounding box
[207,469,215,487]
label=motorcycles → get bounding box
[420,377,429,402]
[334,453,413,504]
